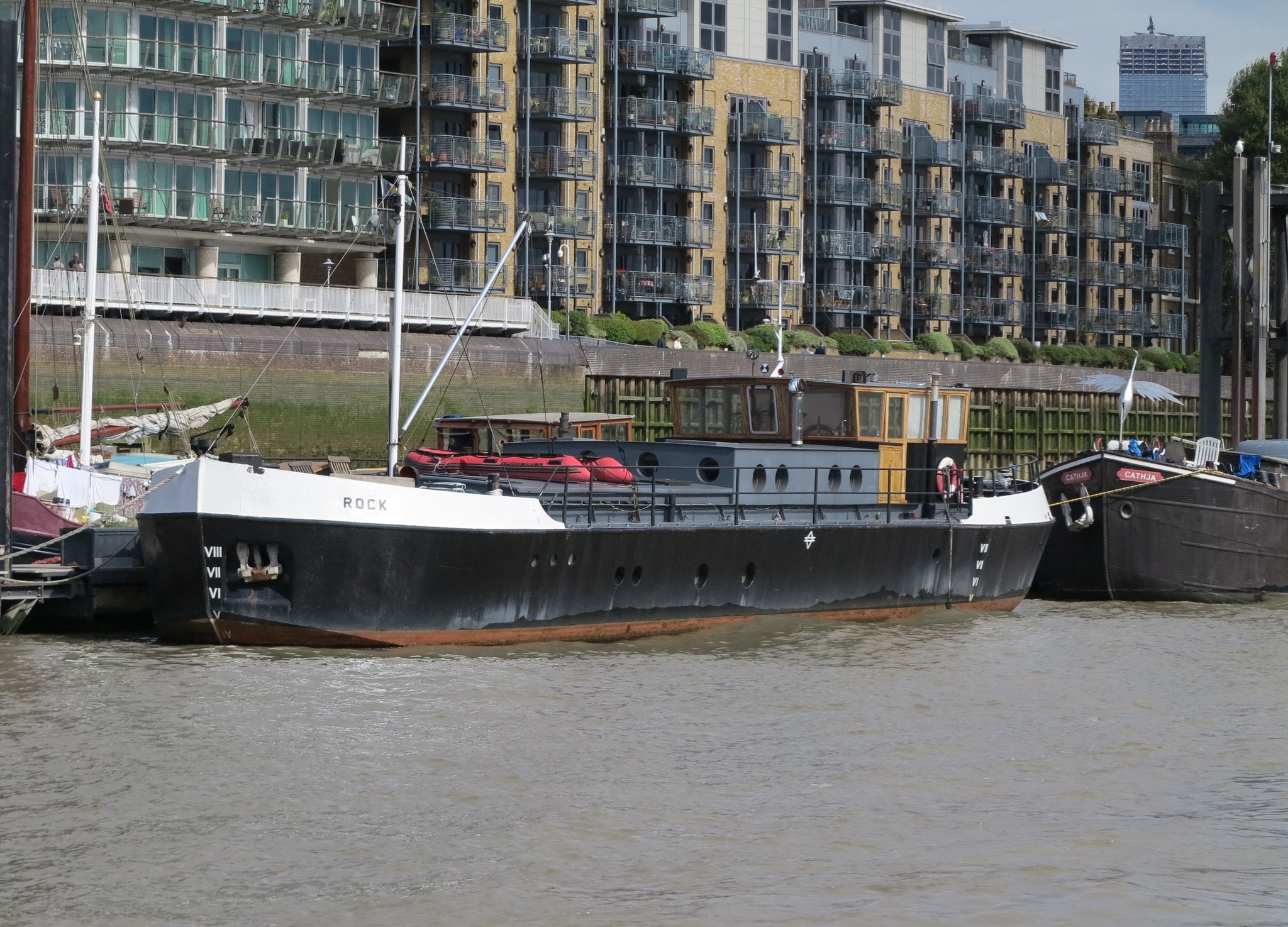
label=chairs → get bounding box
[828,129,846,145]
[564,157,583,174]
[288,455,352,479]
[575,217,590,234]
[694,51,712,75]
[351,214,384,236]
[528,93,548,111]
[132,192,148,216]
[820,288,843,309]
[822,234,856,253]
[1183,437,1220,470]
[1226,454,1261,483]
[210,198,230,222]
[50,188,70,214]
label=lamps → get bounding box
[1065,289,1068,295]
[1020,140,1025,291]
[898,118,904,278]
[1065,337,1068,341]
[954,74,959,81]
[1046,144,1050,149]
[981,80,985,86]
[512,6,518,252]
[948,127,954,334]
[1065,241,1069,247]
[767,99,773,106]
[722,92,730,321]
[813,46,818,52]
[855,53,859,60]
[1066,195,1069,200]
[599,19,607,258]
[799,103,807,324]
[873,114,880,328]
[1087,152,1091,157]
[1070,98,1073,103]
[470,1,479,248]
[1020,333,1024,338]
[928,123,933,129]
[687,89,694,263]
[999,137,1005,289]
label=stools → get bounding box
[579,106,590,116]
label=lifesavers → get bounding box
[936,457,958,499]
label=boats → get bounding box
[135,134,1054,650]
[1034,140,1288,602]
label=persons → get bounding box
[657,332,667,348]
[801,344,811,355]
[68,253,84,272]
[815,342,826,355]
[52,255,65,271]
[673,336,682,349]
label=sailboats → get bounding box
[0,0,249,634]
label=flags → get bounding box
[382,178,412,206]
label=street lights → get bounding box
[558,244,569,340]
[322,259,335,287]
[544,230,557,319]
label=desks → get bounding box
[829,239,850,254]
[232,207,262,225]
